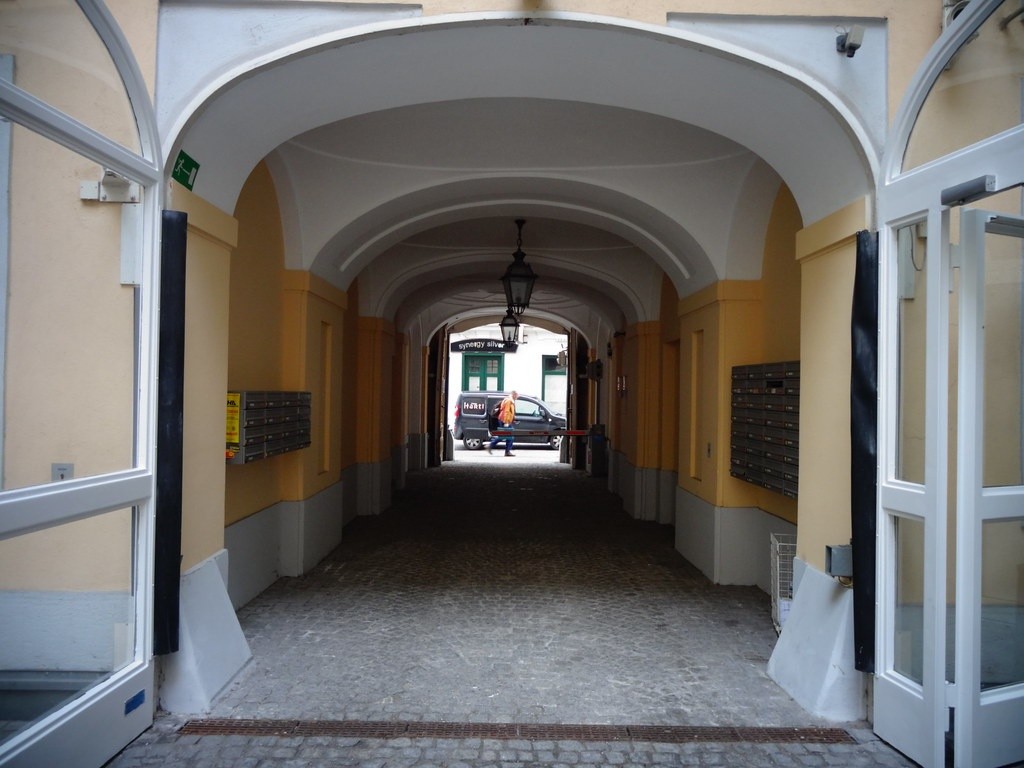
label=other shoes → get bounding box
[505,452,515,457]
[486,446,493,454]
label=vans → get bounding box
[452,390,566,450]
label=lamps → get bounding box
[499,310,519,347]
[501,217,538,315]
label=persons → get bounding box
[484,390,519,457]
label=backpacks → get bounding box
[492,397,513,416]
